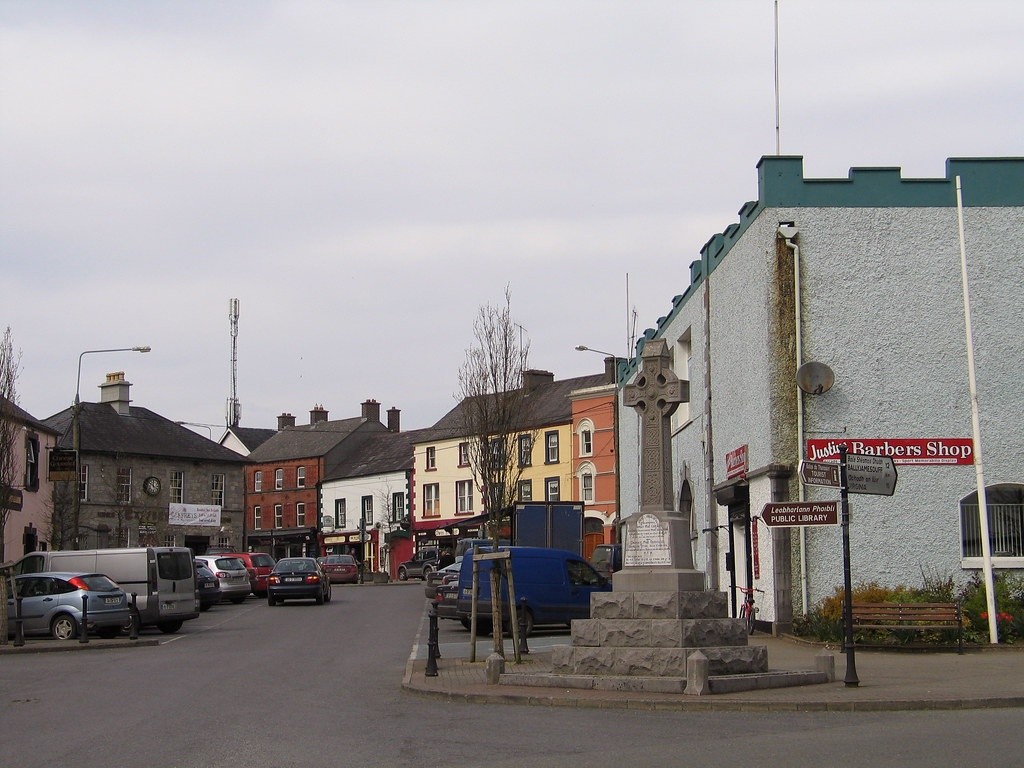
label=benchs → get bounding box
[840,603,963,656]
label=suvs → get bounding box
[397,547,455,581]
[205,544,275,597]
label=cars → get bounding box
[267,557,330,605]
[193,558,222,611]
[194,554,252,604]
[7,572,130,640]
[322,554,363,585]
[435,572,463,620]
[425,555,483,599]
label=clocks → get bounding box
[143,476,161,497]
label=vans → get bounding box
[456,546,618,638]
[7,547,200,634]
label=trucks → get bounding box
[588,544,621,583]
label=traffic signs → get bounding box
[759,502,840,528]
[847,453,898,495]
[799,458,843,487]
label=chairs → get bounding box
[35,581,47,596]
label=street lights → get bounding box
[72,346,151,503]
[576,344,623,536]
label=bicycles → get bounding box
[731,584,764,634]
